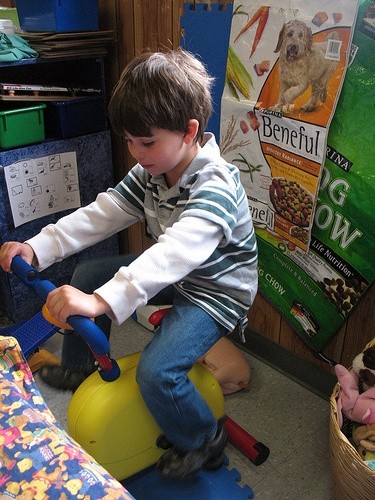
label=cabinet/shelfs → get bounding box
[0,0,123,150]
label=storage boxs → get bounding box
[0,102,47,151]
[15,0,99,32]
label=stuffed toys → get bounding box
[354,423,375,460]
[352,338,375,395]
[334,364,375,429]
[195,334,252,398]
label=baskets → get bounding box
[329,338,375,500]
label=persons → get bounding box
[0,47,259,482]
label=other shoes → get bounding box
[158,424,229,483]
[40,365,85,392]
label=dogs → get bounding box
[268,20,340,115]
